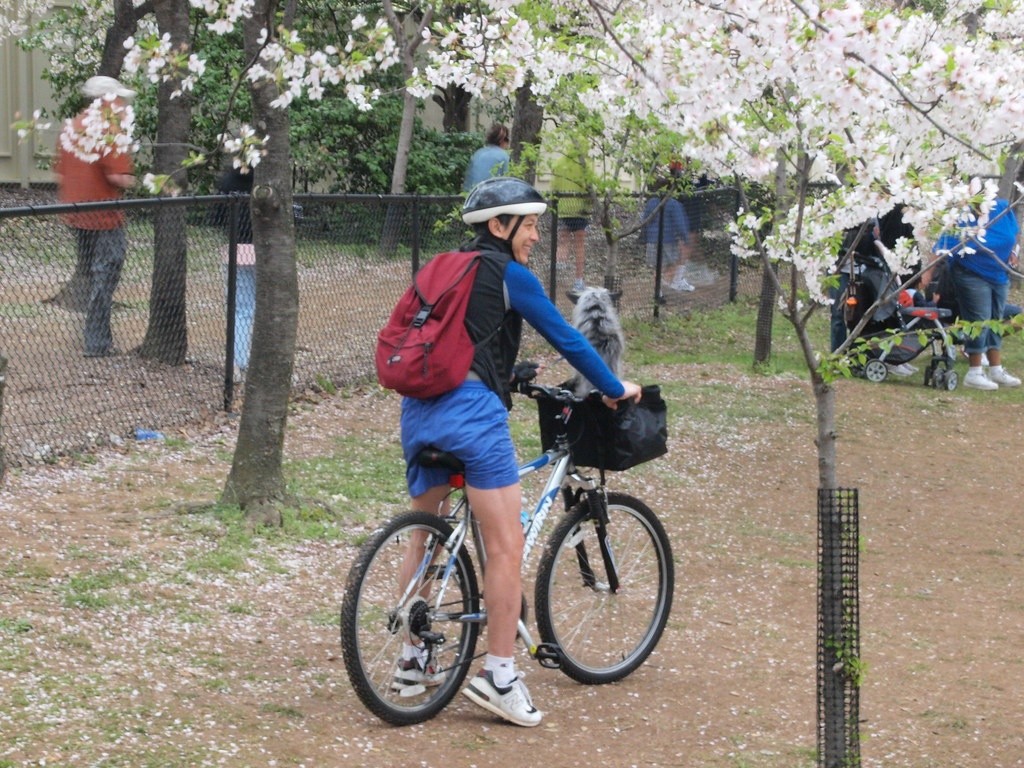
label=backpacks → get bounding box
[374,251,511,397]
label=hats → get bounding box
[82,76,138,98]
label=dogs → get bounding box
[564,286,625,398]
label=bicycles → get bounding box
[340,377,674,724]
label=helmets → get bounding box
[461,177,548,225]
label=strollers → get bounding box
[839,216,960,391]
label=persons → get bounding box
[390,176,650,728]
[641,177,689,299]
[212,160,257,382]
[878,200,923,291]
[919,197,1022,391]
[904,275,940,308]
[61,76,137,358]
[462,124,509,191]
[551,135,592,288]
[828,204,890,370]
[670,149,714,290]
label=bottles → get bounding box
[846,297,857,321]
[520,495,529,529]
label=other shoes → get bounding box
[83,343,122,358]
[566,284,583,303]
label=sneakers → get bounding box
[671,279,695,291]
[988,367,1021,387]
[461,669,542,727]
[884,363,913,376]
[963,373,998,390]
[392,656,448,690]
[903,363,920,372]
